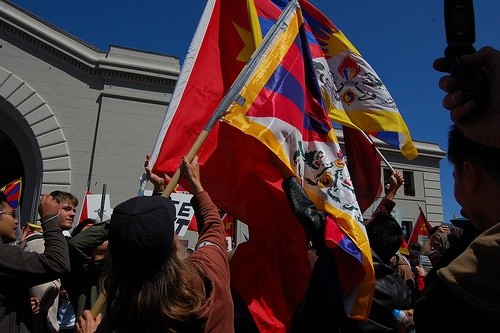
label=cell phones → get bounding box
[444,0,487,108]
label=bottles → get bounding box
[392,309,407,321]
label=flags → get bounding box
[408,212,432,248]
[78,191,92,222]
[0,179,22,210]
[145,0,418,333]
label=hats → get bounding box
[108,195,176,267]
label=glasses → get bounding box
[0,212,16,219]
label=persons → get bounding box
[266,145,415,333]
[75,151,234,333]
[0,191,70,333]
[17,191,81,333]
[364,46,500,333]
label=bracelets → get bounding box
[389,188,396,195]
